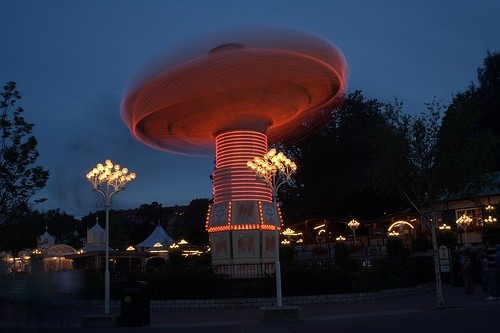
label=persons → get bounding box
[480,240,500,301]
[460,250,473,294]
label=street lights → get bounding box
[247,148,297,307]
[85,159,137,314]
[282,228,295,241]
[455,214,473,244]
[348,219,360,245]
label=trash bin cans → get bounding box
[119,286,155,327]
